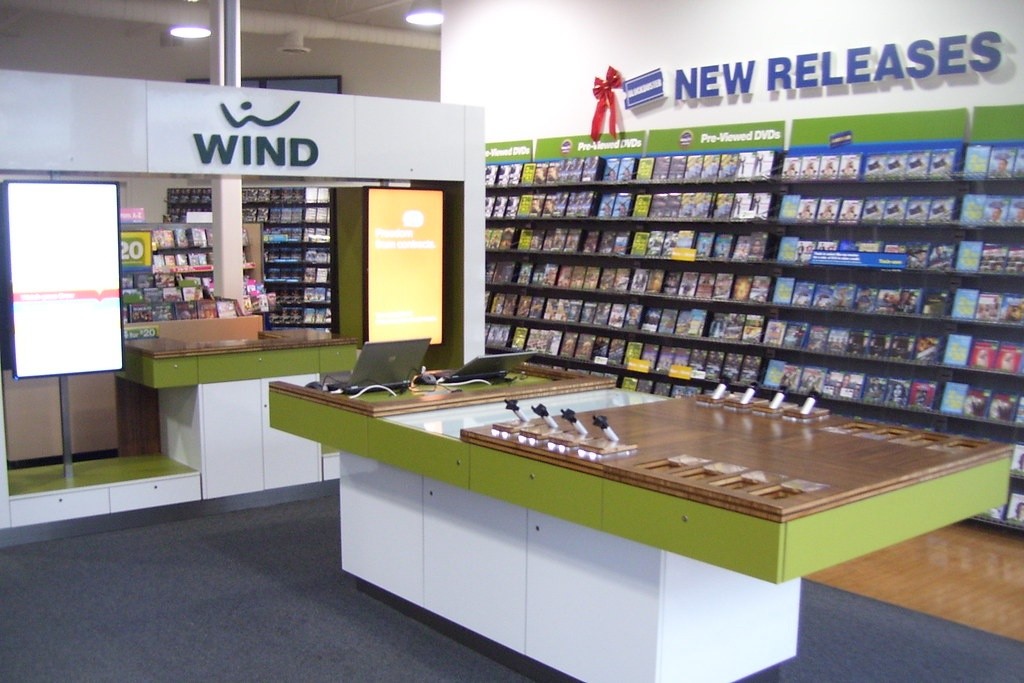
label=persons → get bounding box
[990,207,1002,224]
[996,159,1011,177]
[752,239,762,254]
[892,290,912,312]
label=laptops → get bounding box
[327,337,430,393]
[430,351,538,382]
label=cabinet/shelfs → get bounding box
[124,222,280,331]
[269,389,801,683]
[0,344,358,530]
[166,187,340,334]
[486,142,1024,533]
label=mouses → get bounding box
[414,373,437,386]
[306,382,323,390]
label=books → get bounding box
[167,187,331,329]
[120,228,268,325]
[480,141,1024,531]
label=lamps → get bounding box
[405,0,444,27]
[169,24,214,39]
